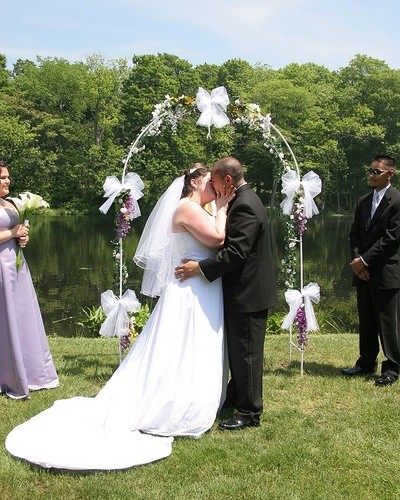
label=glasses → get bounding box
[369,168,389,175]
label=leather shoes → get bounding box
[374,374,397,386]
[217,412,261,431]
[339,364,375,375]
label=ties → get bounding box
[371,192,378,220]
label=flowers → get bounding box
[6,191,50,268]
[115,94,307,349]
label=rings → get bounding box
[181,273,185,276]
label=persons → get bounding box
[341,155,400,386]
[0,161,59,400]
[92,162,238,439]
[173,156,278,429]
